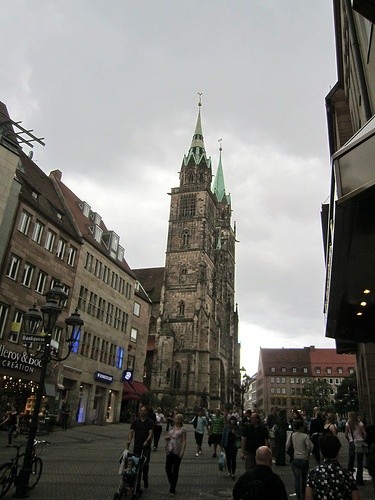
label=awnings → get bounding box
[123,381,150,400]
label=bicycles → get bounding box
[0,436,52,500]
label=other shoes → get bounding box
[153,446,157,448]
[196,451,199,456]
[199,450,202,454]
[348,464,355,472]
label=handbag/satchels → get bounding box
[287,432,294,458]
[354,440,369,453]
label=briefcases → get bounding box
[217,449,226,472]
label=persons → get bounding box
[345,411,367,486]
[126,405,157,489]
[304,434,360,500]
[123,460,137,474]
[233,446,288,500]
[240,413,272,471]
[222,408,289,458]
[220,416,241,481]
[153,407,166,450]
[189,409,212,456]
[0,406,19,447]
[292,407,348,465]
[165,414,187,497]
[207,409,226,458]
[285,418,311,500]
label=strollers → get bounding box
[112,440,147,500]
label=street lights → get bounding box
[12,278,85,500]
